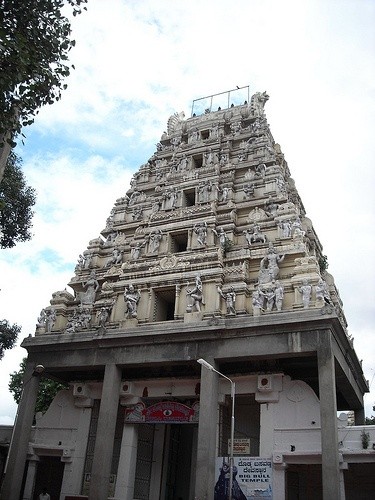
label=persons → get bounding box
[215,462,250,500]
[34,96,348,337]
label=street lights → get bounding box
[196,358,235,500]
